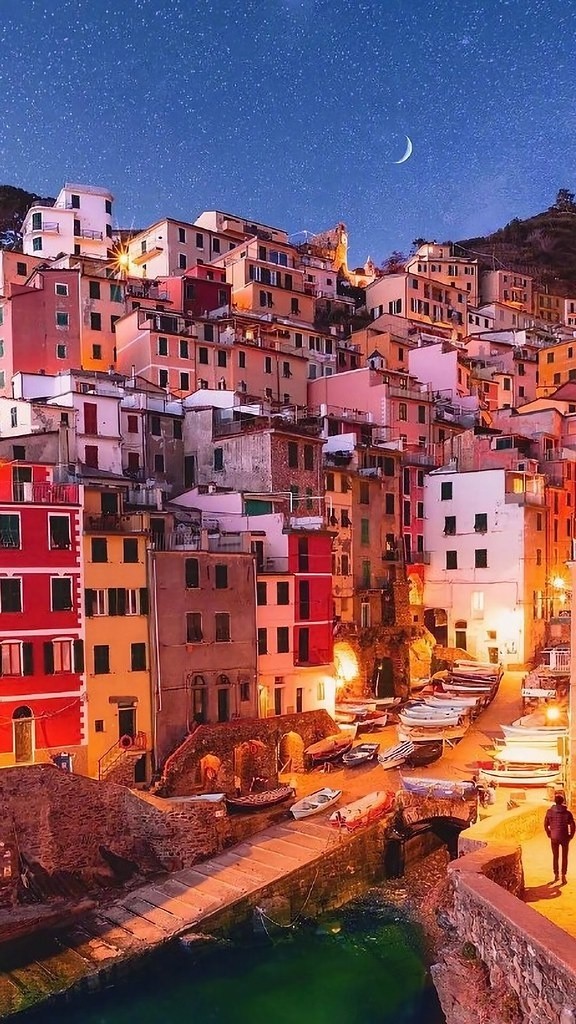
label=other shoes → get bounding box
[555,875,560,881]
[562,876,567,883]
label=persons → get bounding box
[544,795,576,885]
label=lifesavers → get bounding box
[120,735,133,749]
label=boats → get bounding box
[304,731,356,765]
[396,660,504,731]
[289,787,342,820]
[477,767,561,788]
[396,726,466,747]
[486,721,568,771]
[397,770,479,800]
[329,790,393,828]
[342,743,380,767]
[224,786,297,816]
[377,739,415,771]
